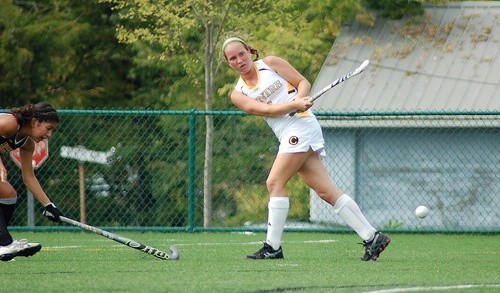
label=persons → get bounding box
[0,102,64,261]
[222,37,391,261]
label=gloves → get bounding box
[42,202,63,222]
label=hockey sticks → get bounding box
[42,209,180,261]
[288,60,368,117]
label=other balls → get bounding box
[415,206,428,218]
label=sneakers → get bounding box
[245,239,283,258]
[356,230,391,260]
[0,238,42,260]
